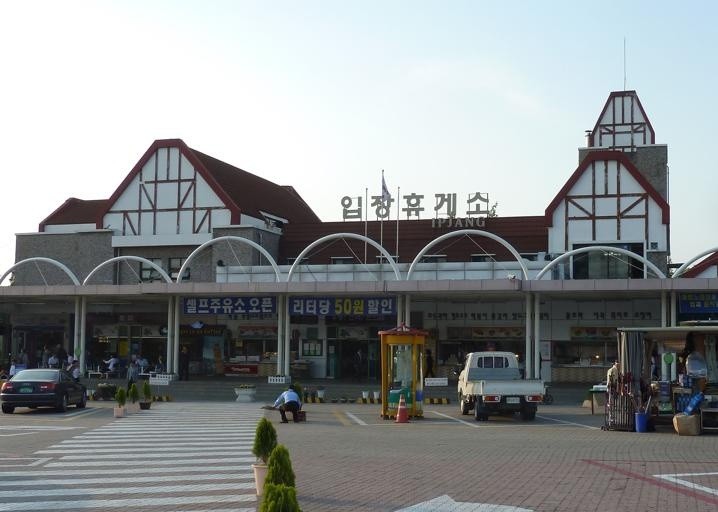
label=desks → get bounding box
[672,387,692,414]
[589,389,606,415]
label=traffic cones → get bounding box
[396,393,409,423]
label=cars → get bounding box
[0,368,87,414]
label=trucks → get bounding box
[454,351,546,420]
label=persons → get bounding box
[177,344,190,381]
[0,343,166,400]
[271,385,302,423]
[351,351,360,378]
[422,349,435,378]
[605,359,617,407]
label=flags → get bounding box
[381,172,391,203]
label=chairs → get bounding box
[7,360,167,379]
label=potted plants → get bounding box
[233,383,257,402]
[361,385,380,400]
[250,419,277,497]
[111,381,156,418]
[316,385,327,399]
